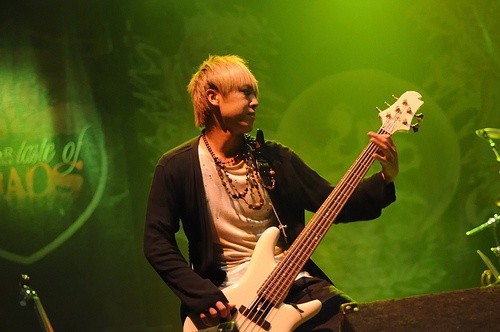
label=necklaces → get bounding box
[200,127,278,213]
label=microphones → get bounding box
[17,273,33,299]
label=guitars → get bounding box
[177,89,423,332]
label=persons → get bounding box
[142,55,399,332]
